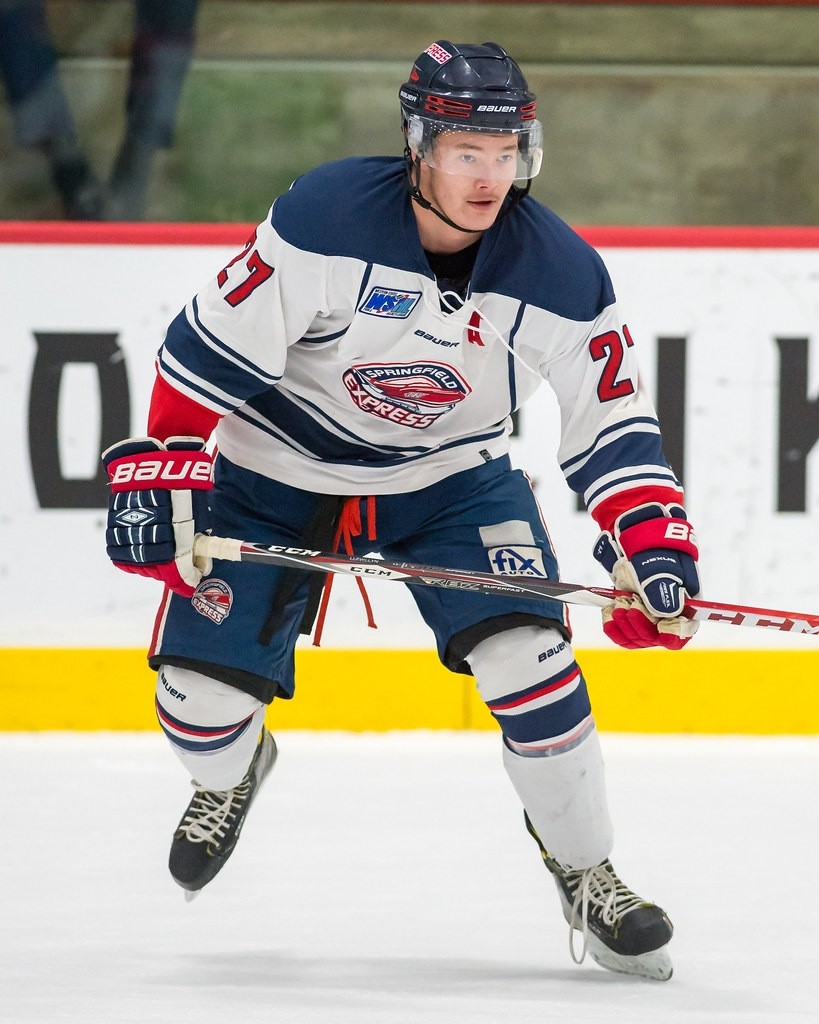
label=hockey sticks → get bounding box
[194,528,819,648]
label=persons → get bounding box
[0,0,196,221]
[101,41,702,981]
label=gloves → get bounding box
[592,503,703,651]
[101,436,215,598]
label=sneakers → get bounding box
[524,810,674,981]
[168,724,277,902]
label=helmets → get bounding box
[398,39,544,233]
